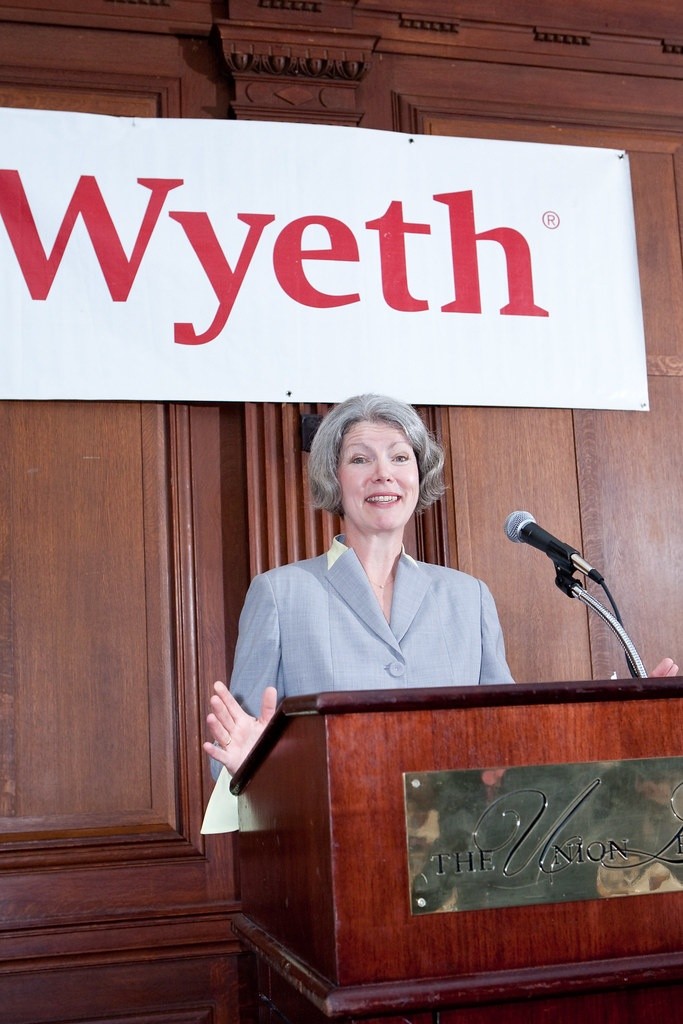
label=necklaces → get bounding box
[370,579,394,588]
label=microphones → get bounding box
[503,511,603,587]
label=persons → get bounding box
[202,394,679,783]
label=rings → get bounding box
[225,738,231,747]
